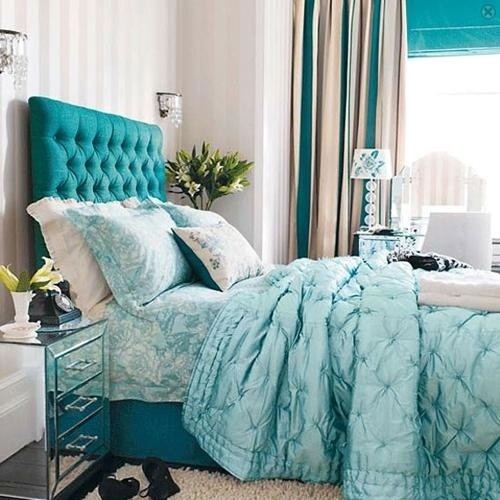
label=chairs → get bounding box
[420,210,493,273]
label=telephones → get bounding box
[28,280,83,326]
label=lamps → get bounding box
[0,29,28,86]
[349,147,394,235]
[157,92,183,128]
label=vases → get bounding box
[0,291,42,339]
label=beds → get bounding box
[27,96,500,500]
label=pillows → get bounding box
[26,195,264,314]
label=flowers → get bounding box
[164,140,256,211]
[0,256,63,296]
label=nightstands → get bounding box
[0,317,112,500]
[358,230,416,258]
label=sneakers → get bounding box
[141,456,181,500]
[97,474,141,500]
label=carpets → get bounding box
[85,450,344,500]
